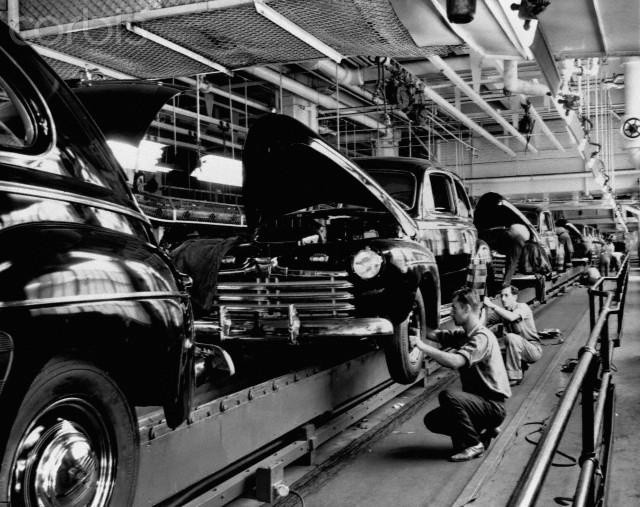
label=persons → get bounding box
[502,224,530,291]
[556,226,575,253]
[483,285,543,387]
[600,238,627,277]
[586,265,601,285]
[409,288,512,462]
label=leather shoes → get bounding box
[509,379,522,386]
[449,427,501,462]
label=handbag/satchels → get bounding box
[537,328,560,339]
